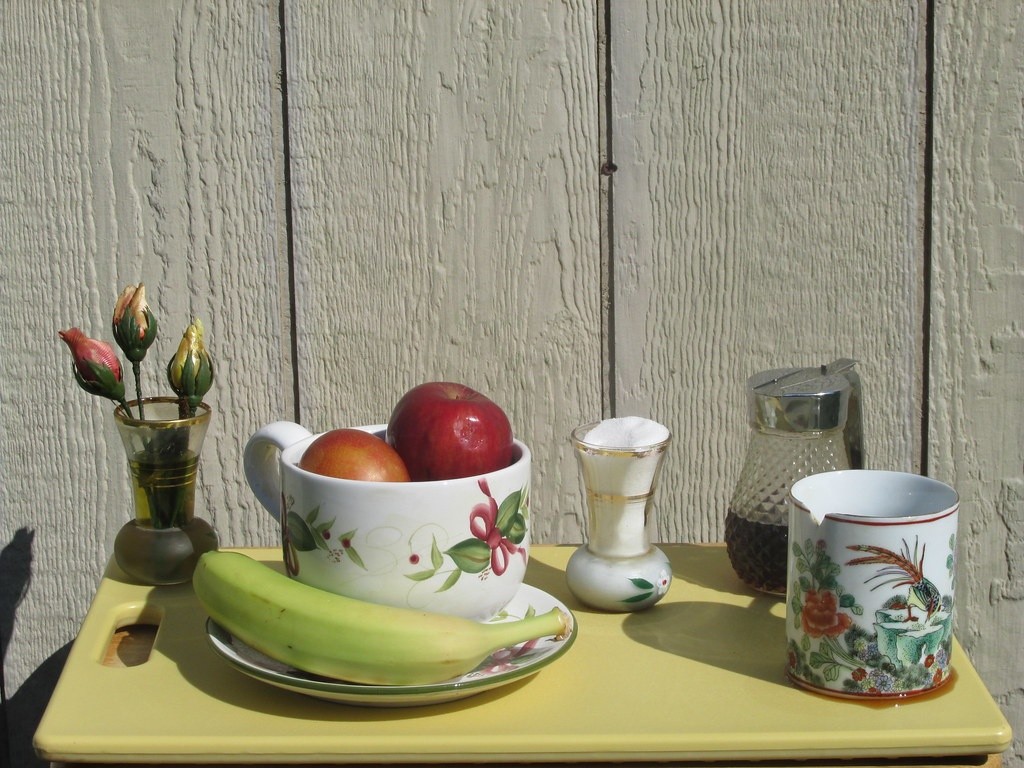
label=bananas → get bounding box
[194,548,566,689]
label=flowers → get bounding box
[56,281,217,532]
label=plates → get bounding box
[203,580,580,710]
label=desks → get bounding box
[30,541,1015,767]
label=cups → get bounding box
[781,467,965,708]
[240,417,536,623]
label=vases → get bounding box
[112,396,222,589]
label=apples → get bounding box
[295,382,513,482]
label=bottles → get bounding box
[565,418,676,613]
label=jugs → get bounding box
[724,360,872,600]
